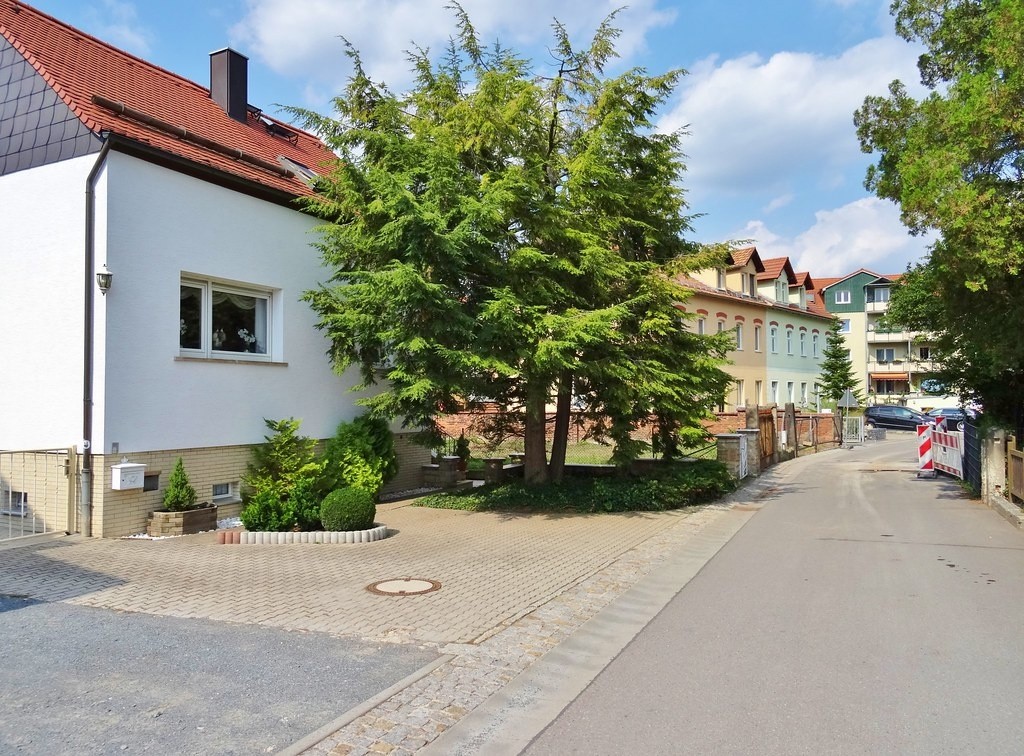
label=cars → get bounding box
[864,404,938,431]
[923,407,984,432]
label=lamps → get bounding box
[95,263,113,297]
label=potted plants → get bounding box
[453,428,470,472]
[143,457,219,535]
